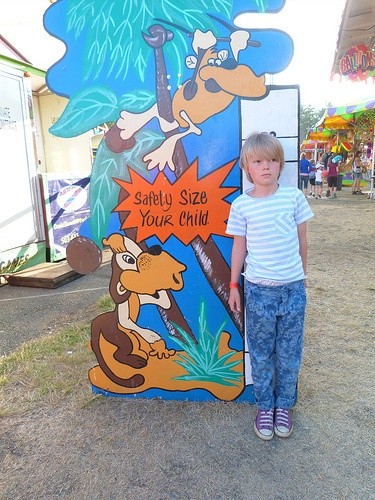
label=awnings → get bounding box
[302,138,329,151]
[306,125,346,142]
[317,96,375,131]
[329,0,375,80]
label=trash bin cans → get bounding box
[335,175,343,190]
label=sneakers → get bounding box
[254,408,274,441]
[275,405,293,437]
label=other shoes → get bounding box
[352,192,357,195]
[319,194,321,198]
[315,196,318,199]
[313,193,314,196]
[310,191,312,195]
[357,191,364,195]
[330,192,337,199]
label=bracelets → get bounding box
[230,283,239,287]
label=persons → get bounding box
[224,133,314,442]
[298,146,364,199]
